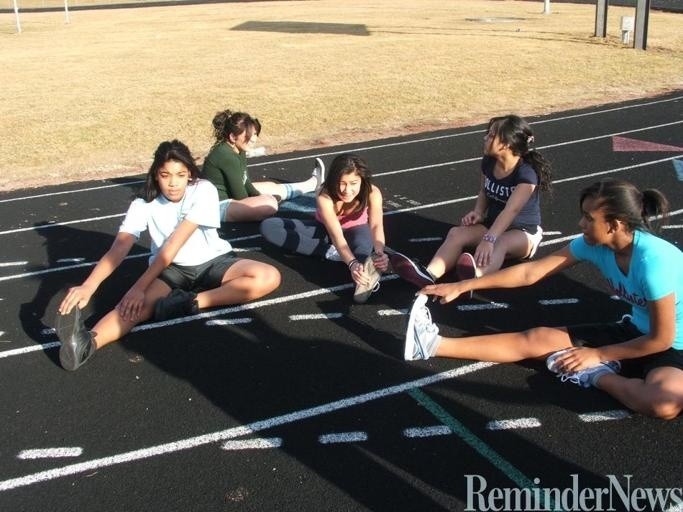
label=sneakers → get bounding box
[389,251,436,289]
[545,346,622,389]
[324,244,344,262]
[152,287,199,323]
[455,252,477,300]
[54,302,98,372]
[353,251,383,304]
[402,293,440,361]
[271,195,281,202]
[310,157,326,192]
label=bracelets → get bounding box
[481,232,497,244]
[348,259,359,270]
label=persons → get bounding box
[389,114,553,290]
[199,110,326,224]
[51,140,283,373]
[258,153,390,303]
[403,176,683,422]
